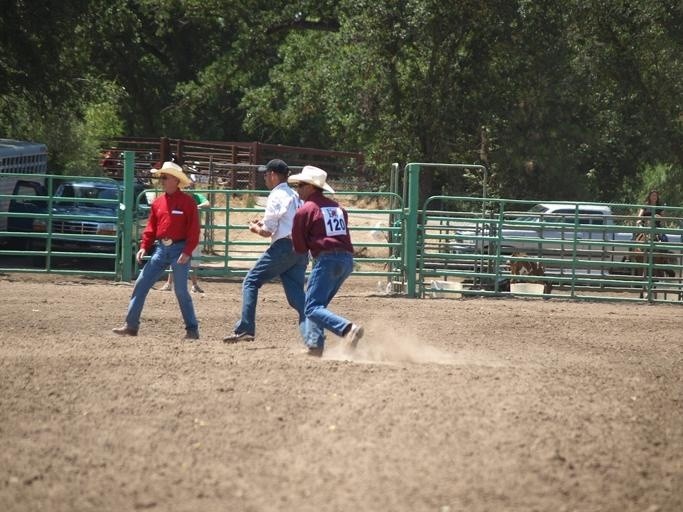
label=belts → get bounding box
[157,238,185,246]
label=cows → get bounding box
[509,254,544,283]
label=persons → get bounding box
[626,189,669,261]
[111,160,201,340]
[220,158,315,346]
[157,176,210,295]
[283,164,365,358]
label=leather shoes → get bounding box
[343,324,364,357]
[179,332,201,340]
[223,331,254,343]
[112,324,138,337]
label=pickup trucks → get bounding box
[452,203,682,275]
[7,176,151,270]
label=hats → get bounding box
[258,158,289,175]
[149,162,191,188]
[288,165,336,194]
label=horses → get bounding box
[633,212,669,302]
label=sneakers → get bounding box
[190,287,204,293]
[159,283,172,291]
